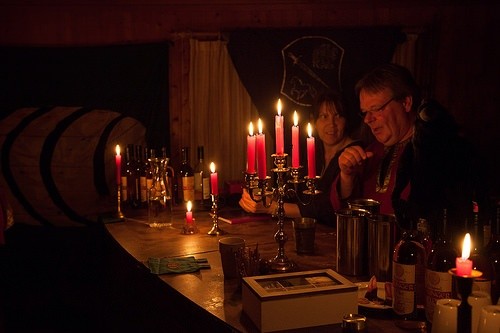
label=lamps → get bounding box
[116,145,122,186]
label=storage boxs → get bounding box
[242,268,359,333]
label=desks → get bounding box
[96,202,500,333]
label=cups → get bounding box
[366,212,398,282]
[469,291,491,333]
[432,299,462,333]
[476,305,500,333]
[218,237,242,275]
[291,217,316,253]
[235,242,261,289]
[334,207,370,275]
[348,199,381,214]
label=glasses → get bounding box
[359,95,400,119]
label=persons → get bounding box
[239,89,366,218]
[330,62,487,247]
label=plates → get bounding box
[354,282,393,309]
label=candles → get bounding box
[210,161,218,195]
[247,98,316,178]
[185,201,193,229]
[456,232,473,277]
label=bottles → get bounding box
[120,143,174,207]
[194,144,212,208]
[178,148,194,209]
[392,202,500,318]
